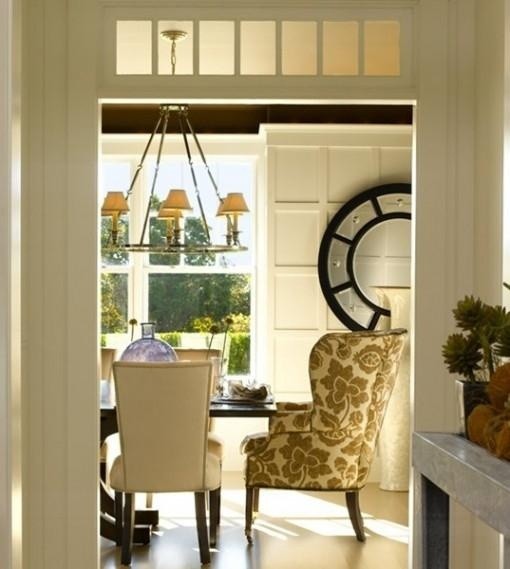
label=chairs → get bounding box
[244,327,409,549]
[174,348,221,432]
[109,361,221,563]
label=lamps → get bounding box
[101,31,247,253]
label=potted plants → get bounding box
[440,296,509,435]
